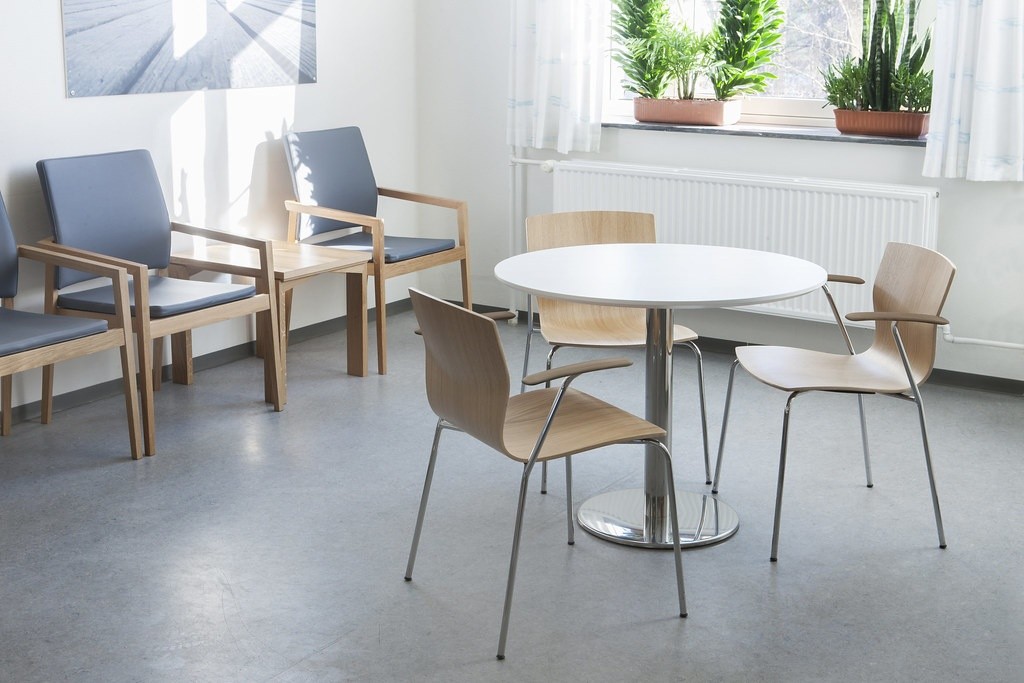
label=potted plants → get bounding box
[818,0,937,139]
[610,0,785,126]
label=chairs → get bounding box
[712,241,957,562]
[37,149,284,455]
[284,127,473,375]
[404,285,687,663]
[0,191,144,459]
[519,210,711,495]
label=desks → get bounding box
[494,244,827,551]
[170,239,373,403]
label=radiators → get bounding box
[553,160,939,330]
